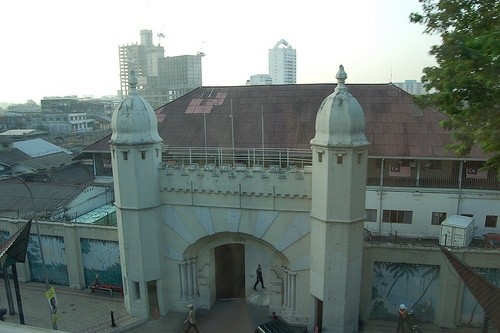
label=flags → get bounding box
[44,285,60,323]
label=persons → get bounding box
[182,303,200,333]
[252,263,267,291]
[89,273,102,293]
[268,312,279,321]
[50,297,57,314]
[395,303,409,333]
